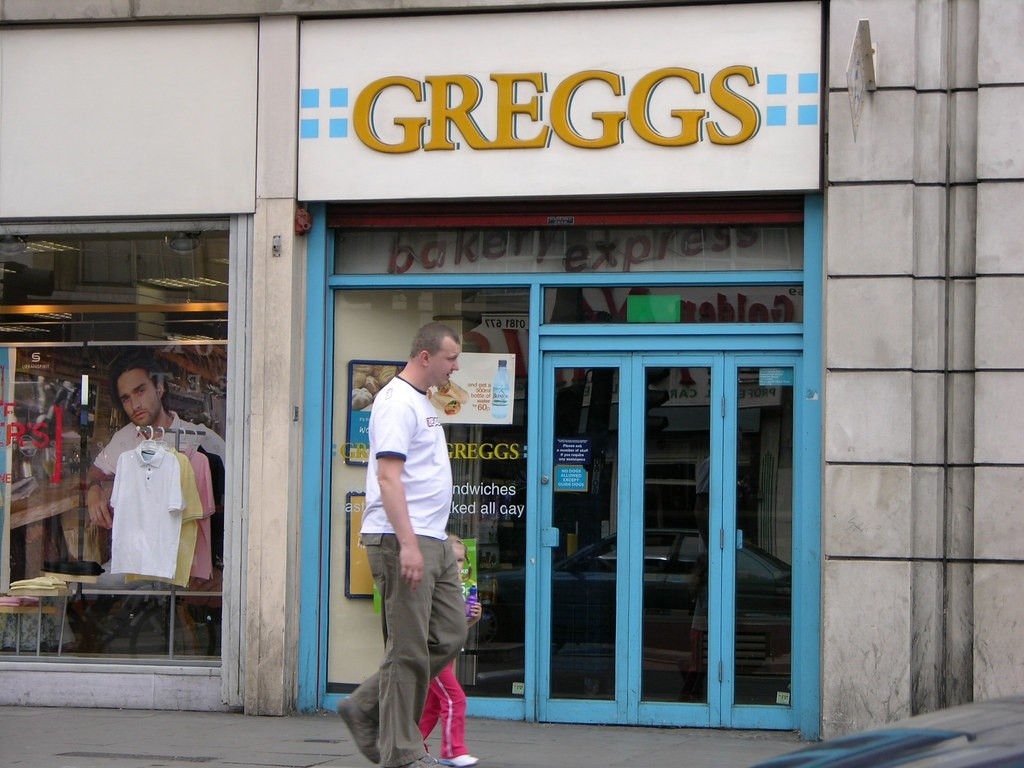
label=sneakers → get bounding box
[337,696,381,764]
[402,754,445,767]
[439,755,478,767]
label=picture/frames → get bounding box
[344,492,375,599]
[346,360,406,465]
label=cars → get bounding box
[452,525,793,691]
[750,690,1024,768]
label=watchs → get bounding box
[85,479,103,491]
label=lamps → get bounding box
[22,238,82,254]
[31,312,73,320]
[167,335,213,340]
[1,325,49,332]
[138,274,229,290]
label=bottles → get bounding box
[464,584,478,617]
[490,359,509,419]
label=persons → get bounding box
[678,567,708,700]
[415,533,483,768]
[335,320,470,767]
[84,359,225,530]
[695,424,742,553]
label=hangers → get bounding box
[120,424,176,467]
[182,430,220,467]
[137,426,187,466]
[166,428,206,468]
